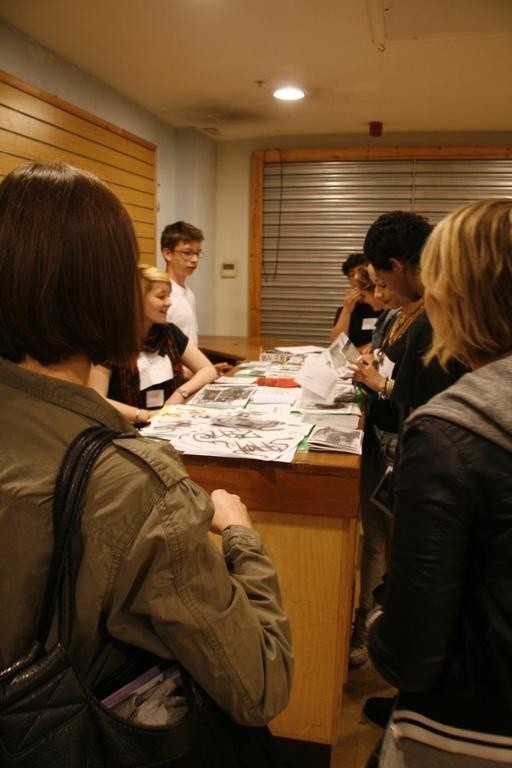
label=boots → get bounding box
[349,608,371,666]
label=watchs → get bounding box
[178,389,189,399]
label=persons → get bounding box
[1,164,290,768]
[86,263,221,433]
[330,198,510,767]
[160,219,235,382]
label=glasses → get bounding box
[168,246,206,261]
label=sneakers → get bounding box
[363,697,394,727]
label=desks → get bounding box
[138,335,367,746]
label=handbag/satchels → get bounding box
[1,644,286,767]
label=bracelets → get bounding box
[136,407,144,423]
